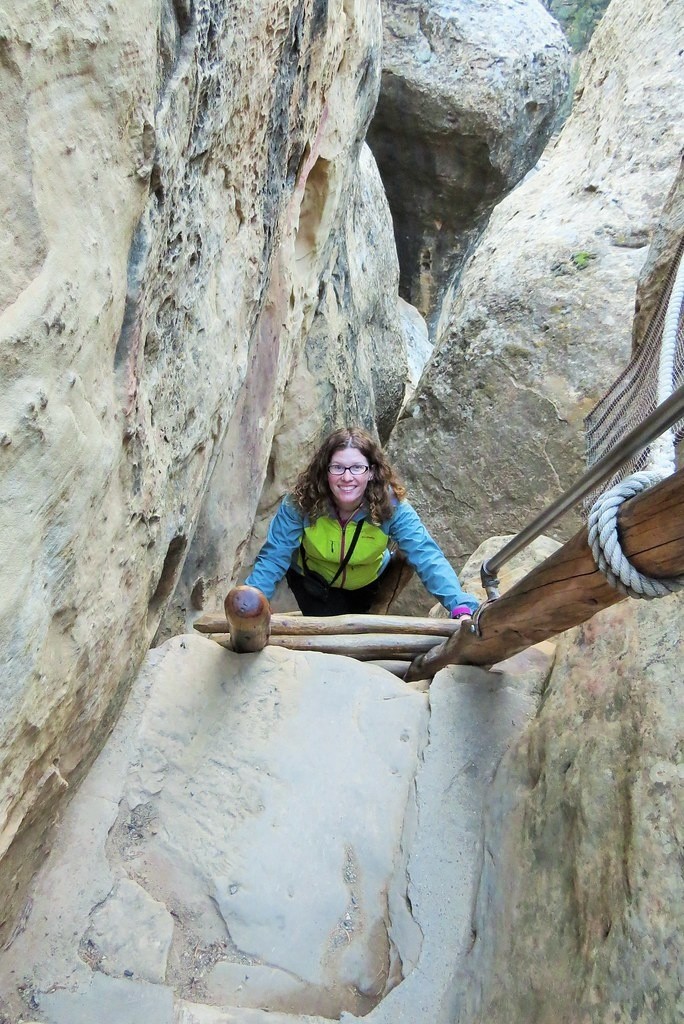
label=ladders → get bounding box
[192,465,684,683]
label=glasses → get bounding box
[327,464,369,475]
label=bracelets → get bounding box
[454,613,465,619]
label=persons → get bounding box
[245,427,479,621]
